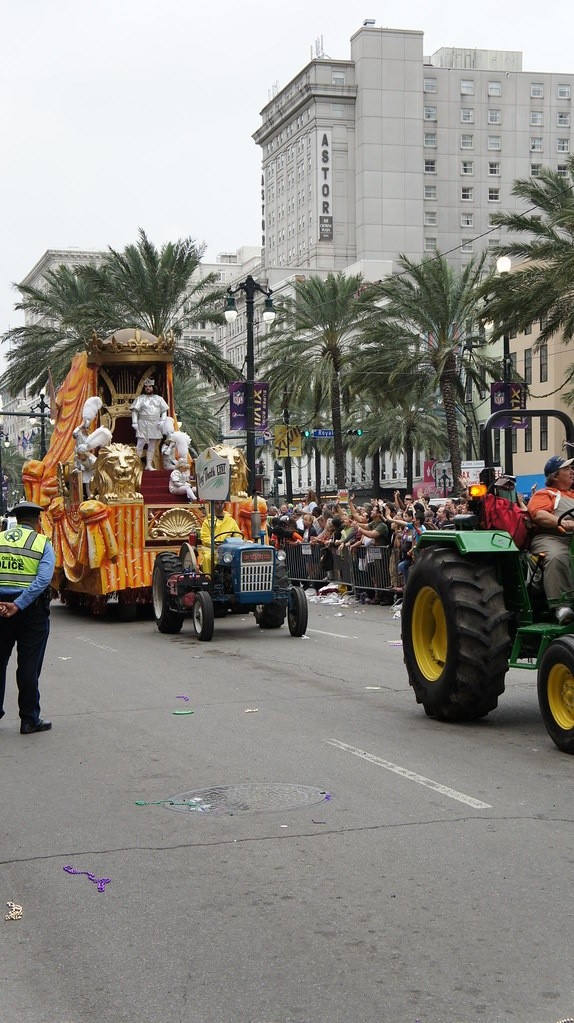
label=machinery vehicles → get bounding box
[150,490,309,642]
[398,406,574,758]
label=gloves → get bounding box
[157,421,164,429]
[132,423,138,430]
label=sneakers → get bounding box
[21,718,51,734]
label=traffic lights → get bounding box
[300,430,311,438]
[273,462,283,486]
[345,429,362,436]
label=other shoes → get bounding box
[145,465,156,471]
[556,606,574,625]
[294,585,395,605]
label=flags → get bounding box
[28,429,36,442]
[22,437,28,448]
[18,436,21,445]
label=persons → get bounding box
[266,488,472,606]
[130,378,170,471]
[518,482,537,510]
[162,434,178,471]
[0,503,56,734]
[169,459,197,504]
[527,455,574,626]
[200,501,242,582]
[74,445,97,501]
[72,419,90,454]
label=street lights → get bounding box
[482,257,515,481]
[30,387,55,462]
[0,426,11,516]
[437,462,454,497]
[221,276,277,497]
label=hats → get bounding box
[164,417,175,441]
[77,426,112,451]
[82,397,102,427]
[173,431,191,467]
[10,501,45,516]
[544,456,574,477]
[144,378,155,386]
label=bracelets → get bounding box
[357,527,361,531]
